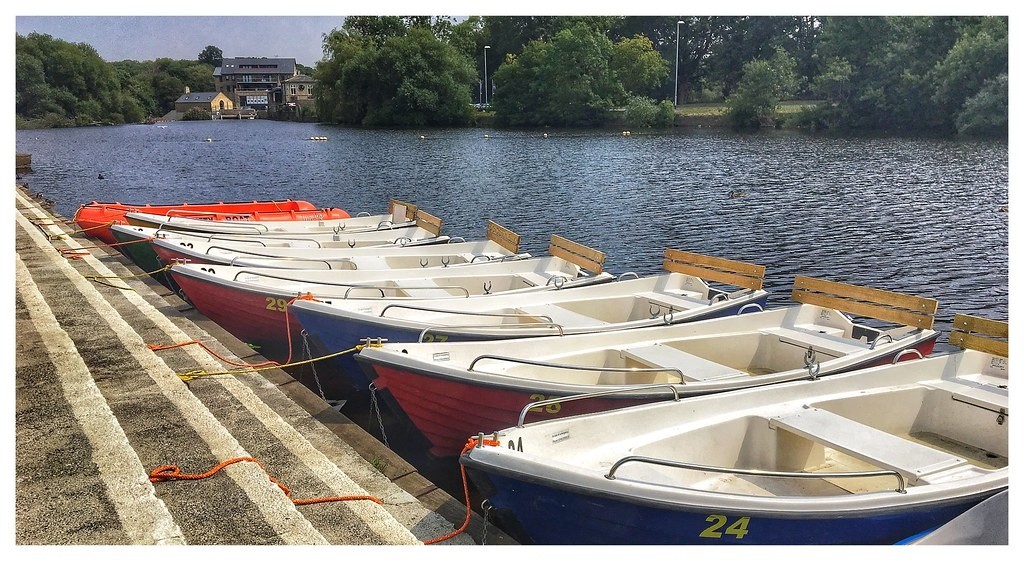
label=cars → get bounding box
[282,102,296,110]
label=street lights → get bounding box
[484,46,490,108]
[674,21,685,106]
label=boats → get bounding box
[352,274,942,451]
[166,233,617,357]
[460,314,1009,545]
[150,220,532,267]
[108,199,450,284]
[285,249,769,402]
[892,490,1008,546]
[74,200,351,250]
